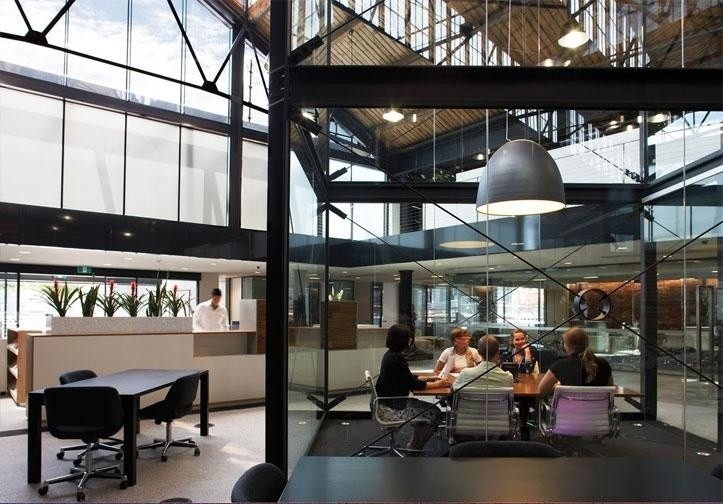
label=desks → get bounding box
[28,370,210,487]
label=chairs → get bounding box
[55,371,124,466]
[137,373,200,461]
[38,386,128,500]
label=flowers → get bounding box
[41,275,192,317]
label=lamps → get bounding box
[382,108,405,123]
[557,0,589,50]
[476,107,566,215]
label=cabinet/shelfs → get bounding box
[6,329,41,405]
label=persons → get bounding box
[500,330,537,375]
[434,327,484,444]
[192,288,229,332]
[539,328,615,427]
[413,304,417,347]
[450,334,513,437]
[373,324,449,457]
